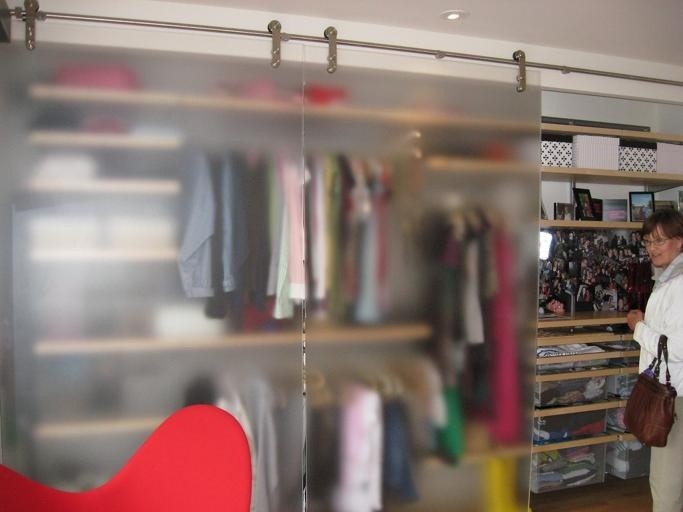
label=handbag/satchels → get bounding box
[623,334,677,449]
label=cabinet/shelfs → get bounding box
[20,83,683,458]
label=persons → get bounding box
[539,198,652,314]
[627,206,683,512]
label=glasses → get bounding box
[640,232,680,247]
